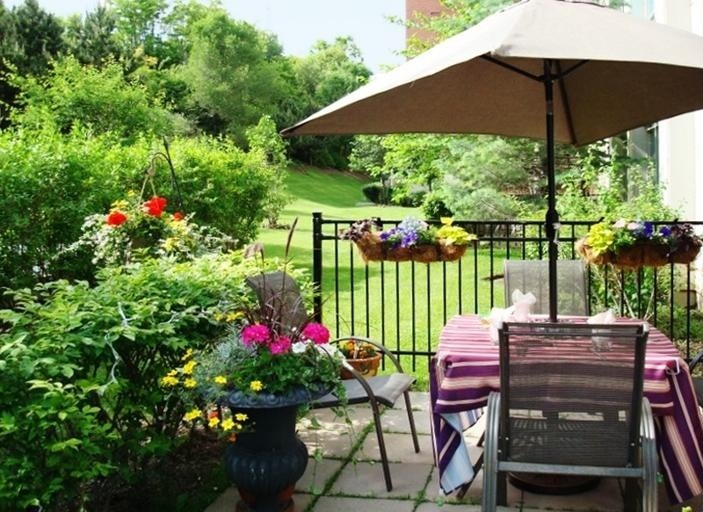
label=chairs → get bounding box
[504,258,589,314]
[482,322,657,512]
[689,349,703,407]
[245,270,420,493]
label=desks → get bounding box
[428,315,702,507]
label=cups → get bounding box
[592,328,612,352]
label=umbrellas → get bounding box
[277,0,703,452]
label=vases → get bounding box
[338,353,382,380]
[575,237,701,265]
[205,380,333,512]
[358,241,467,263]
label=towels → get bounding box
[488,290,535,344]
[586,309,617,351]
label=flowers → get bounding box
[581,217,691,257]
[339,338,375,358]
[338,216,480,250]
[162,219,365,512]
